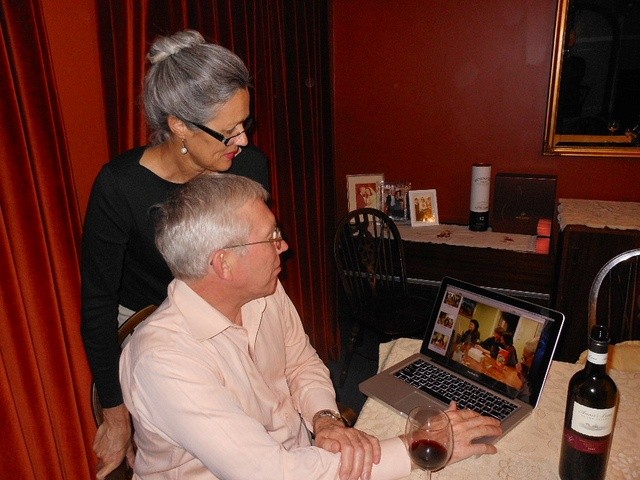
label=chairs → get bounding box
[332,209,437,387]
[90,304,356,479]
[575,248,640,370]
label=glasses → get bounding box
[210,227,283,266]
[161,111,254,146]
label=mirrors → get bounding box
[541,1,640,158]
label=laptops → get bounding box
[358,275,565,448]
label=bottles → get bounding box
[558,324,617,480]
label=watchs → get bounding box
[312,409,342,423]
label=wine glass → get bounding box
[405,406,452,480]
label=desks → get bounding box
[554,197,639,343]
[349,219,554,316]
[338,338,639,480]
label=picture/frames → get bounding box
[345,173,385,225]
[384,182,413,228]
[408,189,439,227]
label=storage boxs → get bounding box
[491,172,557,235]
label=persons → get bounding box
[460,306,518,367]
[385,189,405,217]
[357,182,377,215]
[119,174,502,480]
[413,195,433,221]
[79,30,270,479]
